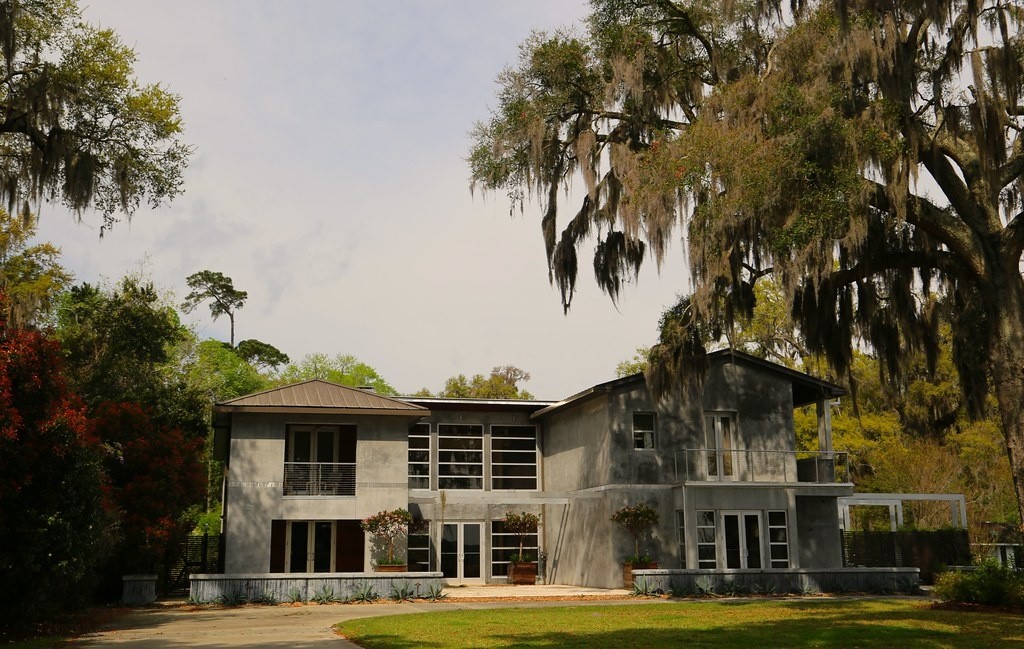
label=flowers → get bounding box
[609,503,662,562]
[498,513,544,561]
[359,507,414,563]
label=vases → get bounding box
[621,560,658,590]
[378,564,408,572]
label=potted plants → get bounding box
[507,560,539,585]
